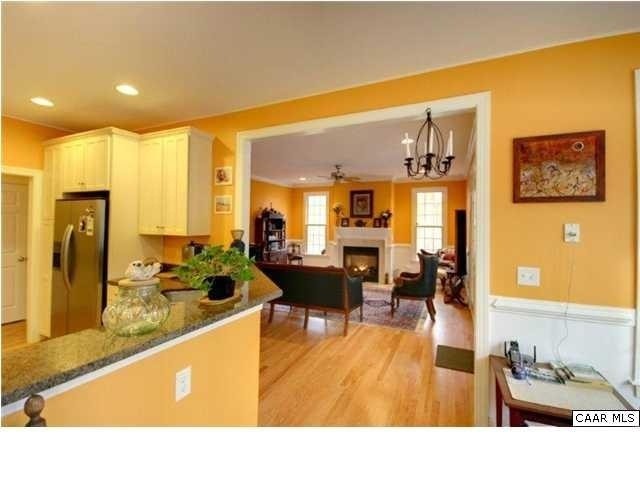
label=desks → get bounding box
[443,269,469,307]
[489,354,636,427]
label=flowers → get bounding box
[333,202,345,215]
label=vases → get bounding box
[230,230,246,256]
[336,216,341,227]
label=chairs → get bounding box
[286,242,303,265]
[390,247,437,322]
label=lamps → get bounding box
[401,106,455,180]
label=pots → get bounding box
[182,241,203,262]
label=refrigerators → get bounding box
[50,197,107,334]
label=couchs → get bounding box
[435,247,455,270]
[253,262,365,336]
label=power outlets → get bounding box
[563,223,581,243]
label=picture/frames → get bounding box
[349,189,373,218]
[214,166,233,187]
[512,129,606,204]
[342,218,348,226]
[374,218,381,228]
[214,194,233,214]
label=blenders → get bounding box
[229,230,244,253]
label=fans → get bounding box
[315,163,361,185]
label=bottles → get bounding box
[507,341,521,368]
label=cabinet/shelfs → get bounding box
[60,124,141,192]
[137,125,214,237]
[454,207,468,277]
[254,206,286,264]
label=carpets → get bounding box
[264,285,427,333]
[435,344,473,374]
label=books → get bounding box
[549,358,610,390]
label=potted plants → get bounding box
[171,244,257,301]
